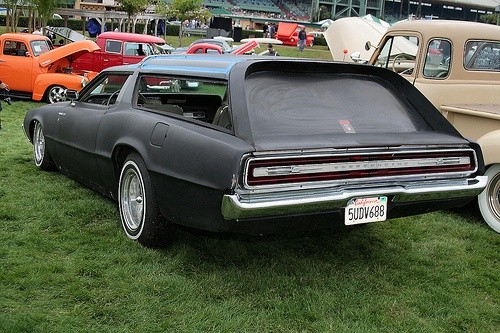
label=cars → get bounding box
[273,21,314,48]
[322,13,500,236]
[22,52,488,251]
[0,27,259,104]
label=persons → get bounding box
[33,26,41,34]
[262,22,275,39]
[185,19,206,27]
[115,26,118,32]
[440,44,450,65]
[298,27,306,51]
[268,44,279,56]
[0,80,6,128]
[268,12,297,21]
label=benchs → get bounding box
[182,27,206,36]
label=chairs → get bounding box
[212,105,230,128]
[137,49,145,56]
[142,97,184,116]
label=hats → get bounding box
[267,43,272,49]
